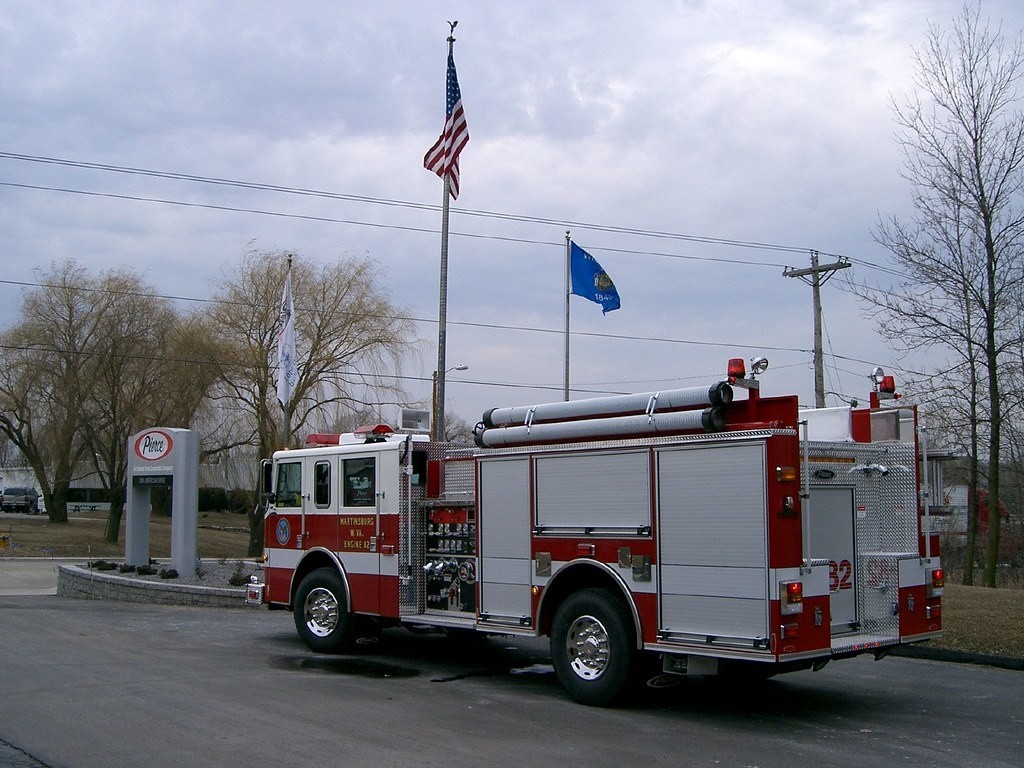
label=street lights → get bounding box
[432,364,469,442]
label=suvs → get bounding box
[2,486,42,514]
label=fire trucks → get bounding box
[248,356,944,705]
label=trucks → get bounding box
[919,487,1010,536]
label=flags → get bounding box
[569,237,621,315]
[276,270,301,405]
[425,53,469,201]
